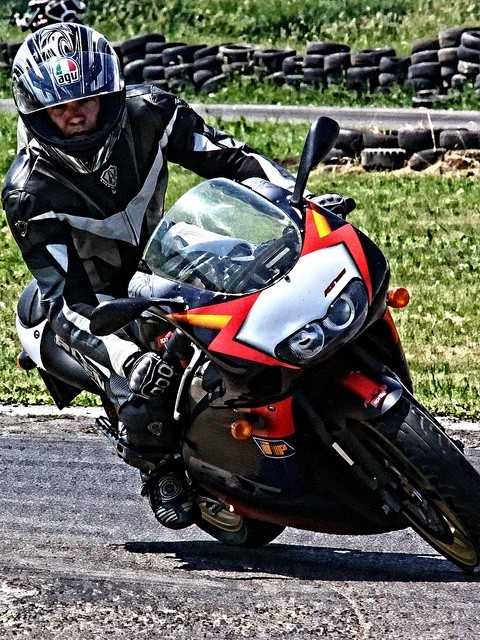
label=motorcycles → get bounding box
[15,116,480,581]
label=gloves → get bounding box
[125,350,176,401]
[309,192,346,221]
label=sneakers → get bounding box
[148,459,201,529]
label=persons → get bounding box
[3,22,345,532]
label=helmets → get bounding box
[11,21,128,175]
